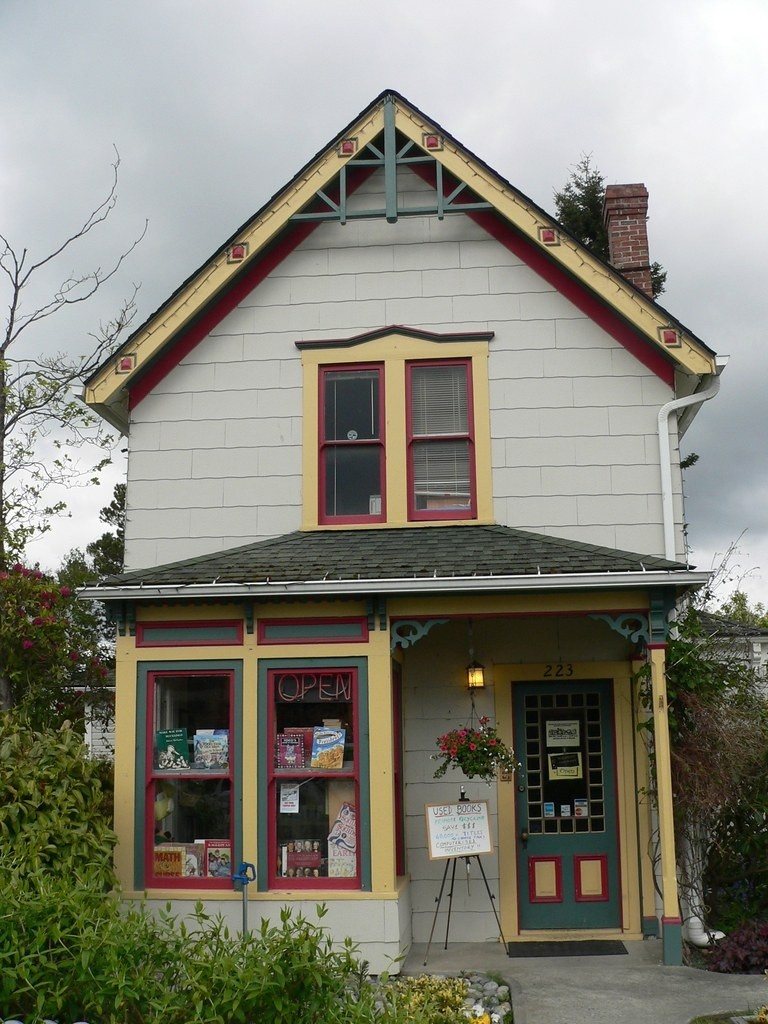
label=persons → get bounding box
[295,841,303,852]
[177,756,186,766]
[313,870,319,877]
[288,869,295,878]
[304,868,311,877]
[296,869,304,877]
[287,840,295,852]
[167,744,180,765]
[312,841,319,851]
[304,840,312,851]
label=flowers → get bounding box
[429,716,525,788]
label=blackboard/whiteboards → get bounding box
[428,800,493,859]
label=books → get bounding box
[152,719,347,878]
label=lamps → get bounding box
[465,660,485,690]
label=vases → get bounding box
[458,756,490,775]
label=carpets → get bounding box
[508,940,629,957]
